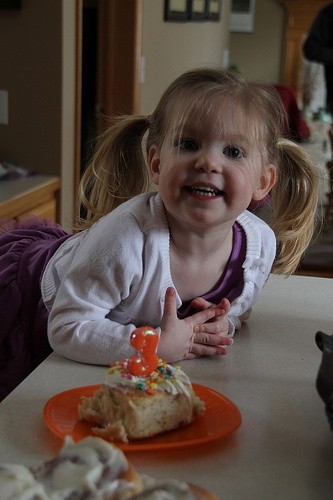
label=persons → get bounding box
[0,68,322,368]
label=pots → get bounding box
[313,329,333,431]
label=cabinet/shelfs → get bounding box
[0,179,62,226]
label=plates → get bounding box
[44,382,241,452]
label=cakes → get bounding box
[78,329,208,441]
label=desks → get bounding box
[1,273,332,500]
